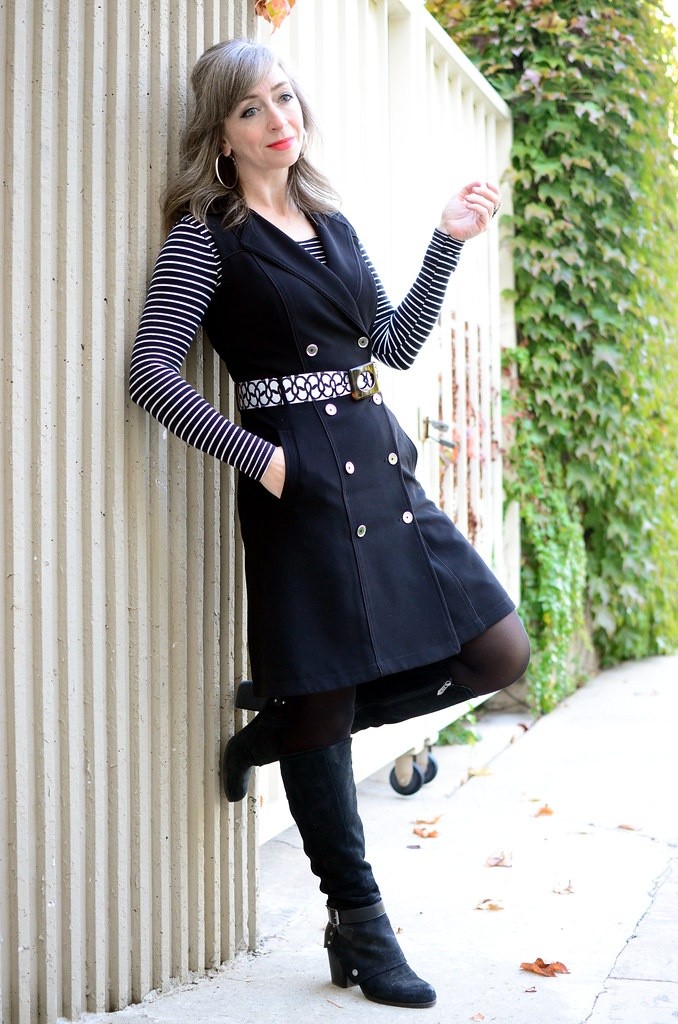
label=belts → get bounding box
[238,362,382,412]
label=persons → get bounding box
[128,38,533,1009]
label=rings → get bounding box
[492,201,502,216]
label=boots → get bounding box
[221,664,478,801]
[277,731,436,1007]
